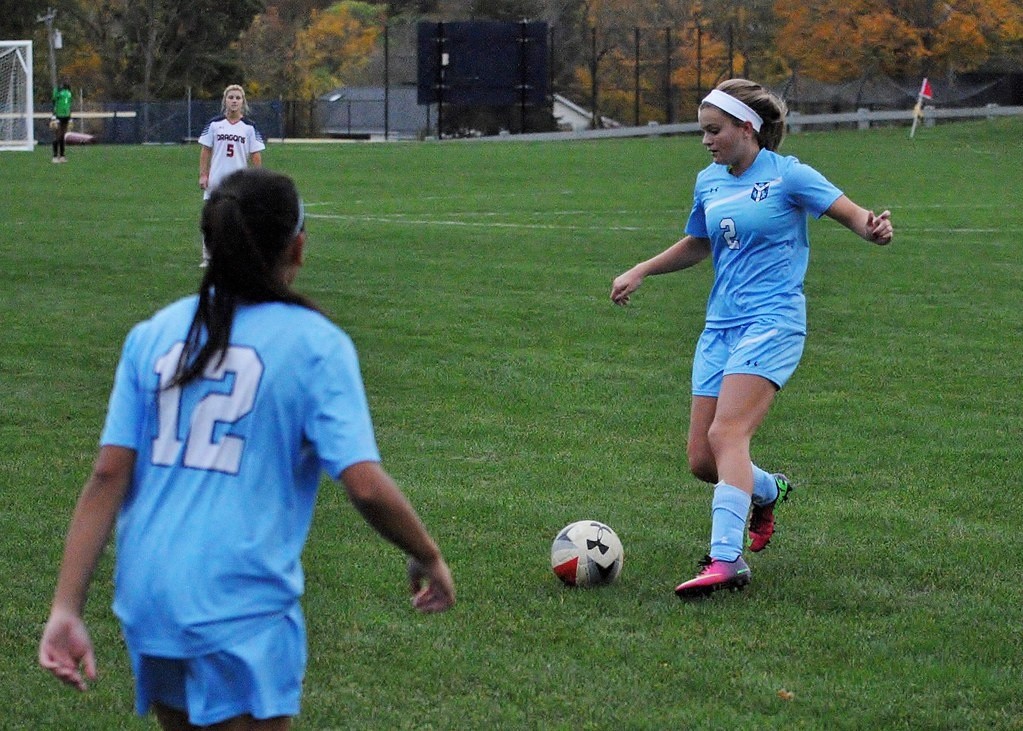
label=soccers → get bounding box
[551,520,623,587]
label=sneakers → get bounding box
[200,260,211,269]
[52,155,67,164]
[676,555,753,601]
[748,472,791,553]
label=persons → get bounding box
[611,76,894,598]
[37,79,466,731]
